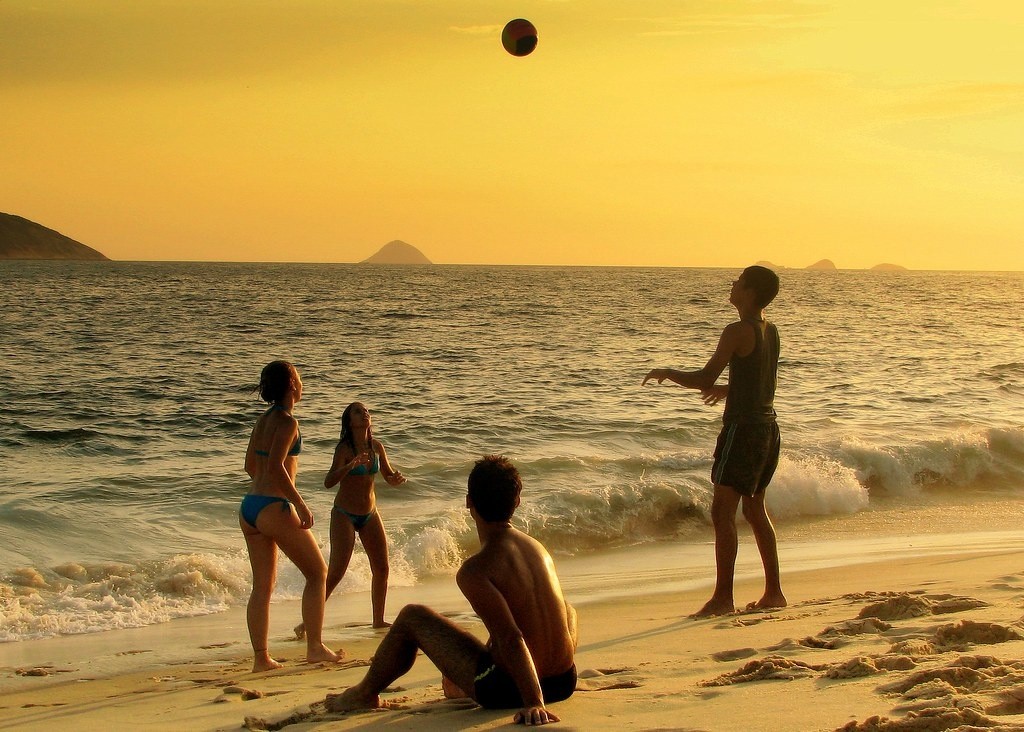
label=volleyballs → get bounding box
[501,17,540,56]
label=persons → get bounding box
[238,361,346,674]
[293,402,408,639]
[642,266,788,617]
[323,453,578,725]
